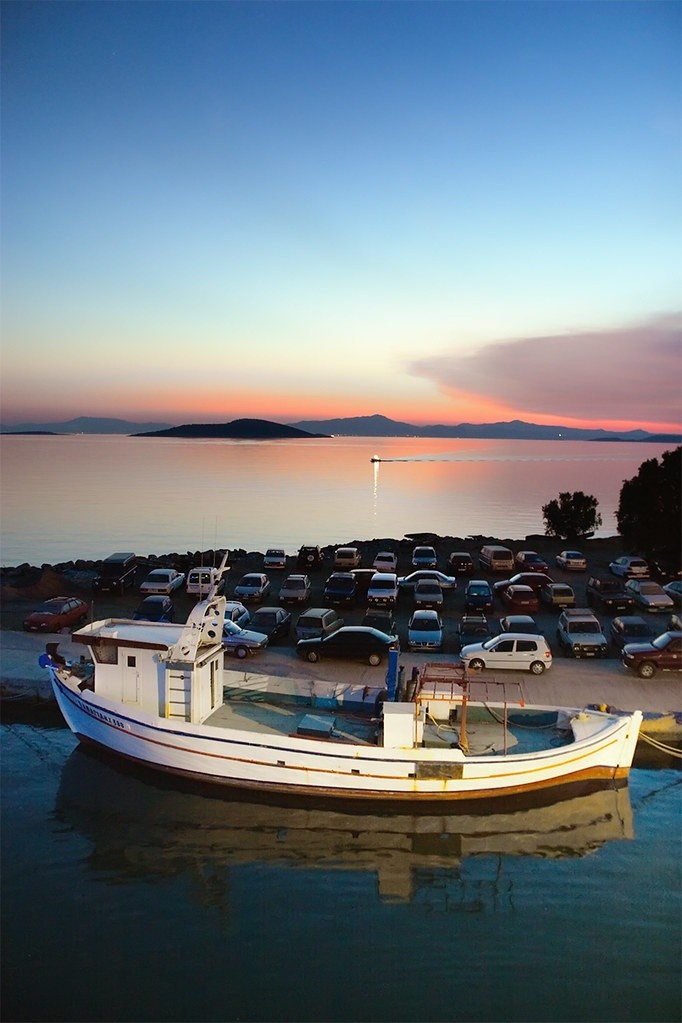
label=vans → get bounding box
[186,566,224,600]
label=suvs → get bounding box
[362,606,398,636]
[322,570,360,606]
[94,551,138,595]
[294,606,345,643]
[205,601,251,630]
[279,574,312,606]
[23,596,89,635]
[132,595,176,623]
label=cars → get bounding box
[407,609,445,653]
[397,569,457,592]
[261,546,682,679]
[242,607,291,645]
[194,618,268,659]
[460,632,553,676]
[413,577,443,611]
[234,572,272,604]
[296,625,402,667]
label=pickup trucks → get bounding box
[139,568,185,598]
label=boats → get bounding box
[46,748,634,949]
[38,511,643,804]
